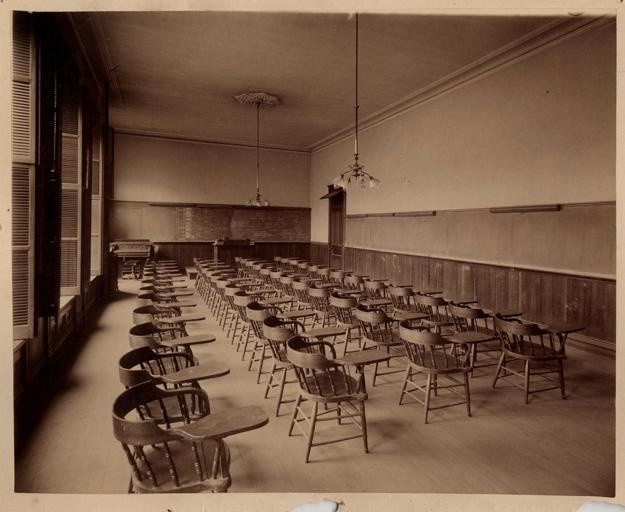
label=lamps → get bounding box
[234,92,280,207]
[331,13,381,192]
